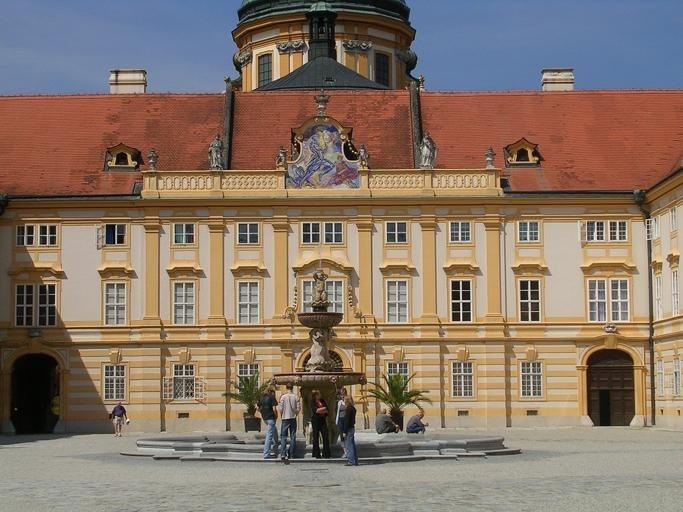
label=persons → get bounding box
[257,384,279,459]
[340,395,359,466]
[146,149,158,161]
[421,131,435,167]
[375,408,400,434]
[406,410,429,434]
[484,146,496,158]
[278,145,288,161]
[335,386,346,458]
[310,269,328,302]
[310,389,330,459]
[277,383,300,461]
[208,134,223,169]
[358,143,366,160]
[110,401,129,438]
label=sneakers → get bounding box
[112,433,122,437]
[262,450,358,467]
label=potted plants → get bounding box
[357,370,434,431]
[221,372,270,432]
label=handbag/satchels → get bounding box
[125,419,130,425]
